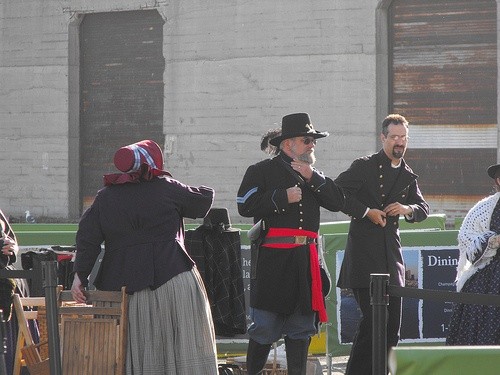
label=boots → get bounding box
[246,338,272,375]
[284,335,311,375]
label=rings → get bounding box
[299,166,300,169]
[393,209,397,212]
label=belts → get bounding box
[261,236,318,245]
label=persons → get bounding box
[333,114,429,375]
[70,140,219,375]
[445,163,500,345]
[0,209,25,375]
[236,113,344,375]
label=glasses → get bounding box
[387,134,411,141]
[299,139,317,145]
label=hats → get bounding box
[104,140,173,186]
[269,112,329,147]
[487,164,500,179]
